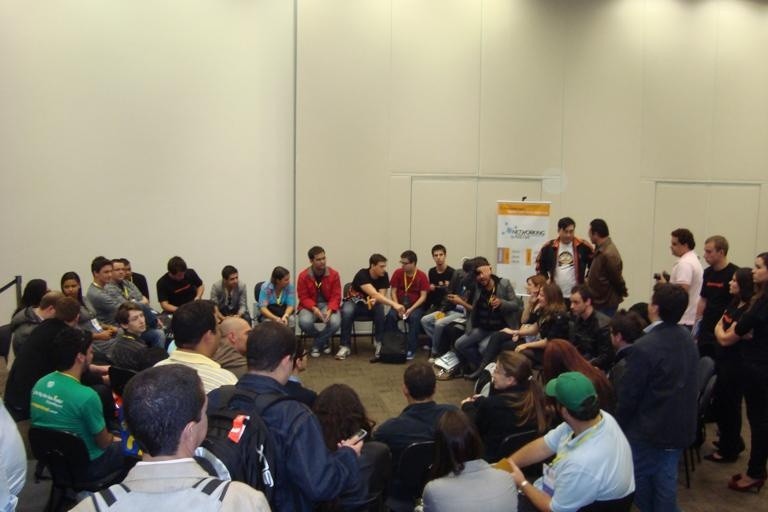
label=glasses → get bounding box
[398,260,411,265]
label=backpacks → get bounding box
[200,385,296,503]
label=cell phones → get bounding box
[348,429,368,446]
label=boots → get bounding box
[463,362,487,382]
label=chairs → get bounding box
[108,366,138,396]
[25,426,122,512]
[253,282,375,355]
[397,425,536,493]
[0,325,15,364]
[680,354,717,489]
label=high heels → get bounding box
[728,472,765,495]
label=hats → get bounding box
[543,371,598,412]
[474,256,490,268]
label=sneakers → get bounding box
[322,341,331,355]
[702,453,735,463]
[310,347,322,358]
[710,439,721,448]
[407,351,415,360]
[373,339,383,358]
[334,344,352,361]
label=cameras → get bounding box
[653,272,670,282]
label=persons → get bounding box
[2,246,635,511]
[607,229,768,510]
[535,217,629,318]
